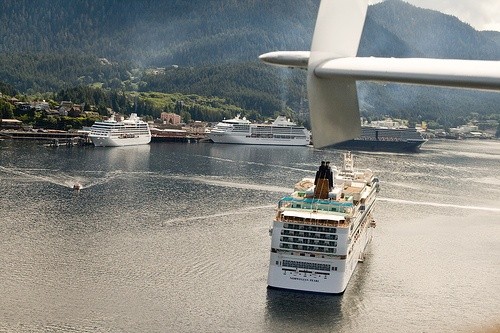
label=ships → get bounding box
[86,112,151,148]
[356,124,429,150]
[205,113,311,148]
[265,149,380,298]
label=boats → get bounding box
[435,125,493,142]
